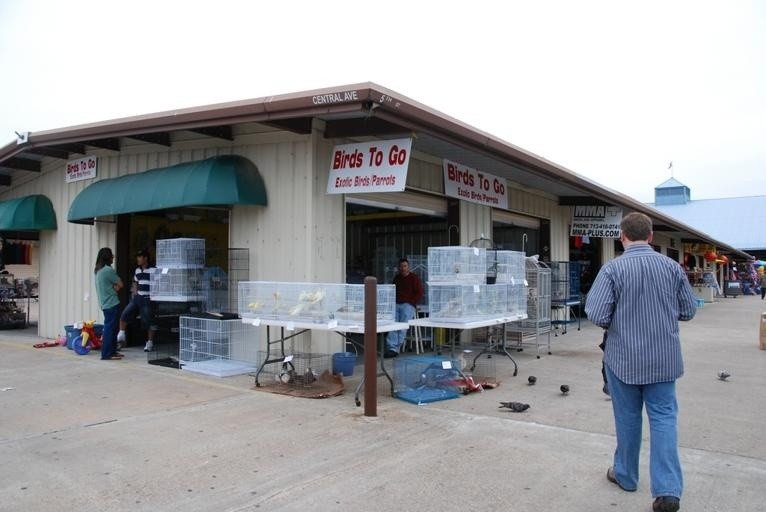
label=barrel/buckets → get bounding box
[333,352,356,376]
[93,323,104,337]
[64,326,81,350]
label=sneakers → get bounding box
[653,495,680,512]
[384,350,398,358]
[144,340,154,351]
[607,466,637,492]
[117,330,125,341]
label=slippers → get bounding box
[110,353,125,359]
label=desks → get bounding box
[241,313,529,406]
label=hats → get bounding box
[134,248,149,257]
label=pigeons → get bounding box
[560,384,571,396]
[527,375,538,386]
[717,369,731,380]
[274,355,318,388]
[498,400,531,412]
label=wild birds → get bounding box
[246,287,335,321]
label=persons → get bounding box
[585,212,698,512]
[384,258,423,358]
[94,247,161,360]
[759,269,766,300]
